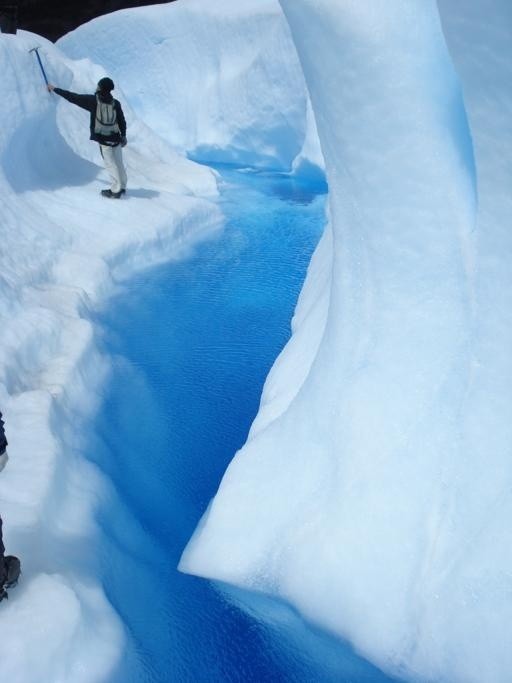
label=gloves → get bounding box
[121,136,127,147]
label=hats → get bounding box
[98,78,114,90]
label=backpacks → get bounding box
[94,93,121,135]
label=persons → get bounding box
[0,410,20,602]
[47,78,127,199]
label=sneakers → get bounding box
[0,552,22,600]
[101,189,125,198]
